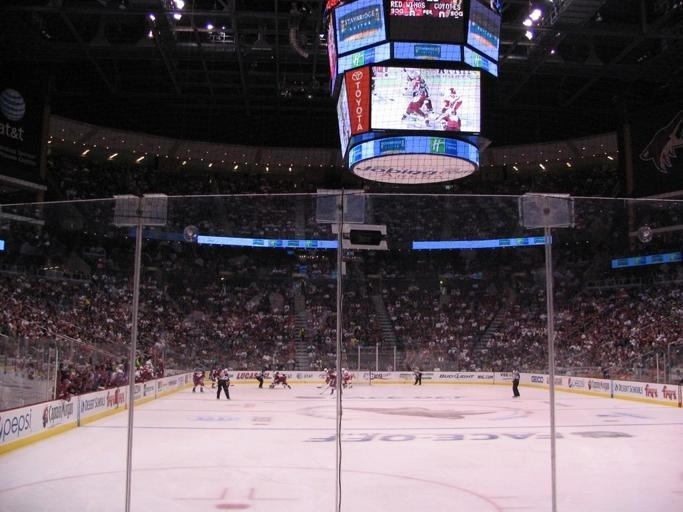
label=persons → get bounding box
[400,68,462,132]
[0,148,683,408]
